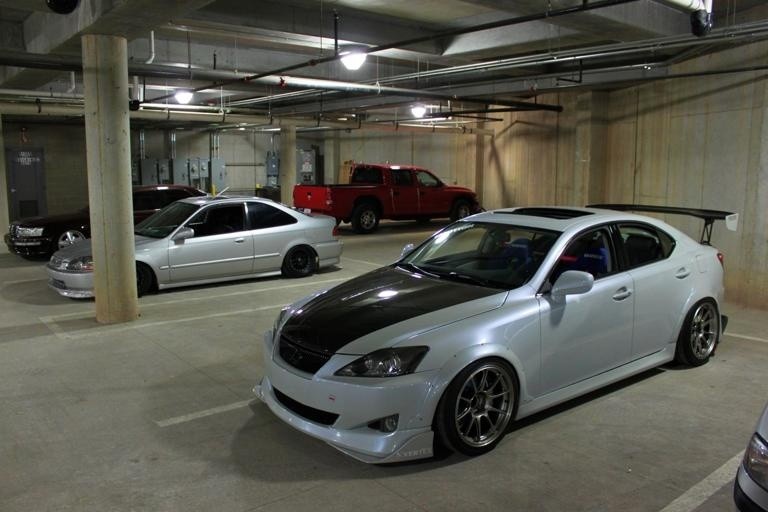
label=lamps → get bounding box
[174,89,193,104]
[338,44,368,70]
[410,104,426,118]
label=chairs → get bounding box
[504,234,657,285]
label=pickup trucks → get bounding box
[288,162,483,237]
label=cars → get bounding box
[245,188,748,470]
[3,181,211,265]
[40,192,347,303]
[729,391,768,512]
